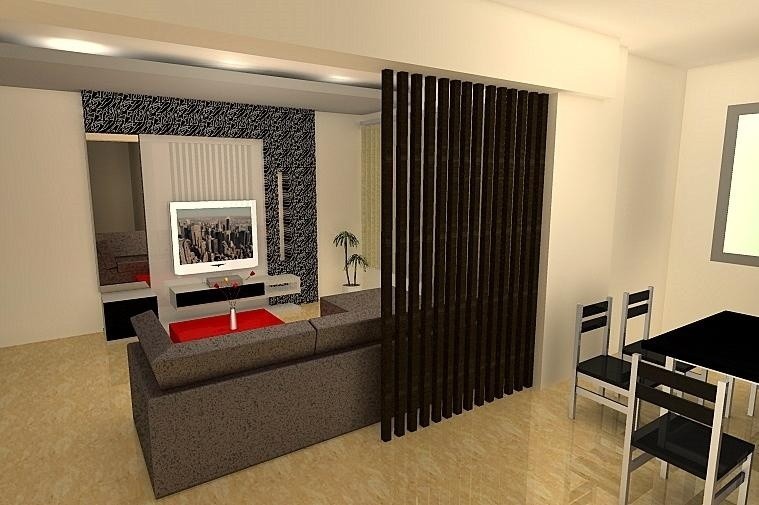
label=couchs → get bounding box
[127,286,422,500]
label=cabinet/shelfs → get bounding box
[169,273,302,323]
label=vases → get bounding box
[230,308,237,331]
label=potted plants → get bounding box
[332,231,368,293]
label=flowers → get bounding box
[214,272,255,308]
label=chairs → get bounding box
[614,287,708,416]
[747,384,759,416]
[619,353,755,505]
[569,296,663,431]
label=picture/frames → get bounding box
[709,102,759,267]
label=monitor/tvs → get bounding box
[169,200,259,276]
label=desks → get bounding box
[642,310,759,462]
